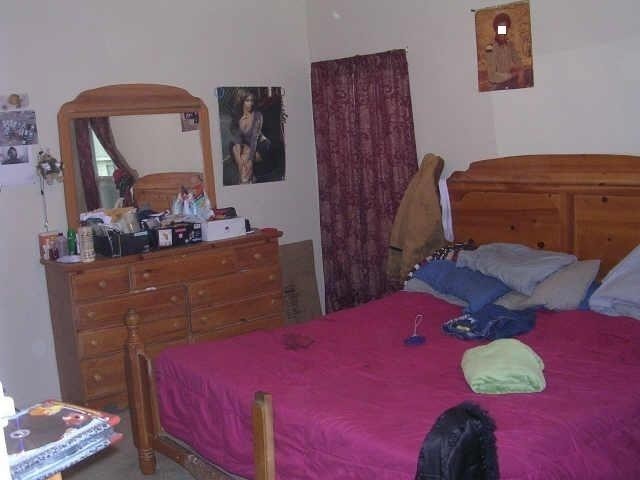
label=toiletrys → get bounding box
[66,226,78,256]
[76,220,97,265]
[55,232,69,258]
[48,241,59,260]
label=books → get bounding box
[1,399,125,480]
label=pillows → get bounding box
[405,241,640,319]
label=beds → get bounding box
[124,155,640,480]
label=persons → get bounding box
[226,85,272,184]
[483,11,528,90]
[1,148,24,163]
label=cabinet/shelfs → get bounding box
[39,229,283,415]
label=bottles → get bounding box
[56,220,96,263]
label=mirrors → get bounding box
[58,84,217,232]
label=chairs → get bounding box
[417,401,499,480]
